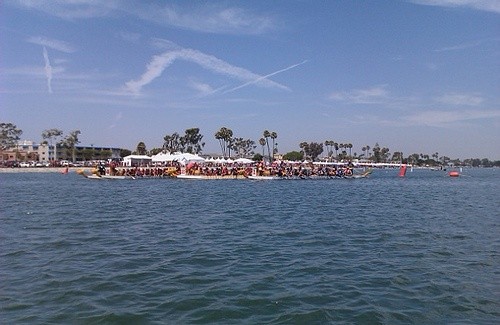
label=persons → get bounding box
[49,155,355,179]
[410,163,466,174]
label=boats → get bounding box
[447,170,460,177]
[89,171,180,180]
[76,167,112,180]
[309,170,373,179]
[169,171,256,180]
[240,171,308,181]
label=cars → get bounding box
[3,158,181,167]
